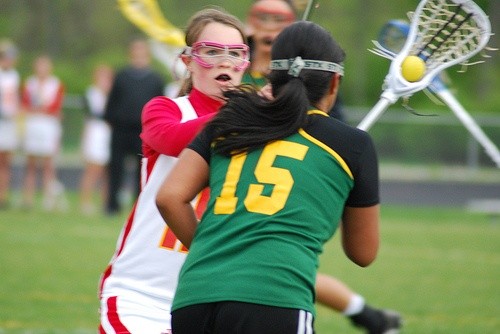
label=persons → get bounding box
[156,22,379,334]
[313,271,403,334]
[99,7,276,334]
[1,0,350,211]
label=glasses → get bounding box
[249,6,294,32]
[184,42,250,70]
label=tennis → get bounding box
[401,54,426,83]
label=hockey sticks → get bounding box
[376,19,500,170]
[357,0,491,133]
